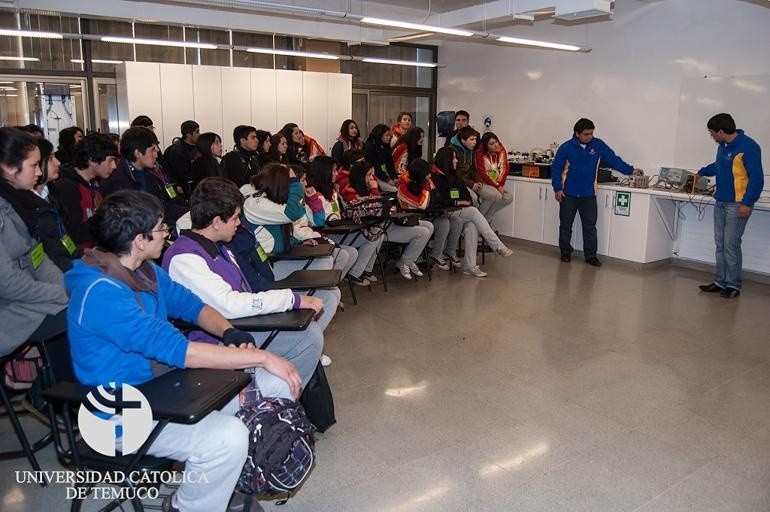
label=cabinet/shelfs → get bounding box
[114,59,194,155]
[251,67,301,143]
[576,185,674,264]
[193,64,251,166]
[513,176,576,252]
[486,175,514,239]
[302,72,353,155]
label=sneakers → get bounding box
[497,246,513,257]
[699,283,722,292]
[585,256,602,267]
[351,272,378,286]
[560,250,570,262]
[431,251,461,271]
[463,266,488,277]
[397,261,423,279]
[720,287,740,298]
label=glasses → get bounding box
[148,224,170,234]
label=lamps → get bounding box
[0,2,592,69]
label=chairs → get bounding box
[0,151,499,512]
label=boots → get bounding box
[23,364,79,431]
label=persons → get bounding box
[0,109,517,512]
[550,118,644,265]
[689,113,765,300]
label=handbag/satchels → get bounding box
[391,213,421,227]
[348,196,385,218]
[235,396,317,494]
[300,359,336,434]
